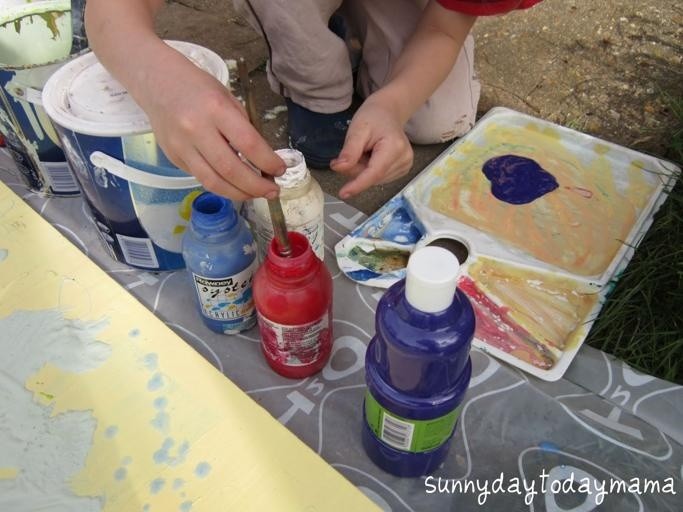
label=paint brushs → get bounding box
[237,58,293,261]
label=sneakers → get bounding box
[285,99,354,169]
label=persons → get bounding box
[84,0,545,201]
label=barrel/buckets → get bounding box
[41,38,242,271]
[0,1,92,200]
